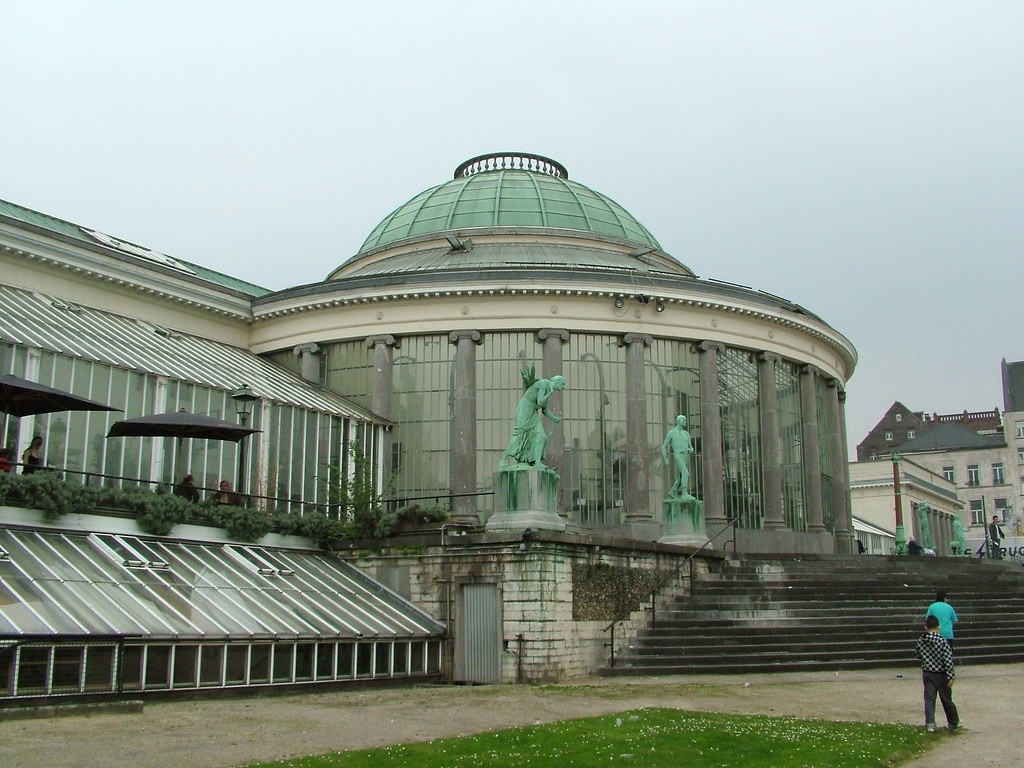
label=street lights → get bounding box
[229,384,262,496]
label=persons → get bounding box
[500,375,566,469]
[924,590,958,653]
[988,515,1006,560]
[662,414,696,500]
[918,501,934,549]
[907,535,924,556]
[915,615,963,733]
[953,513,969,555]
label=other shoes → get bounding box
[928,724,937,732]
[993,556,1004,560]
[948,723,962,730]
